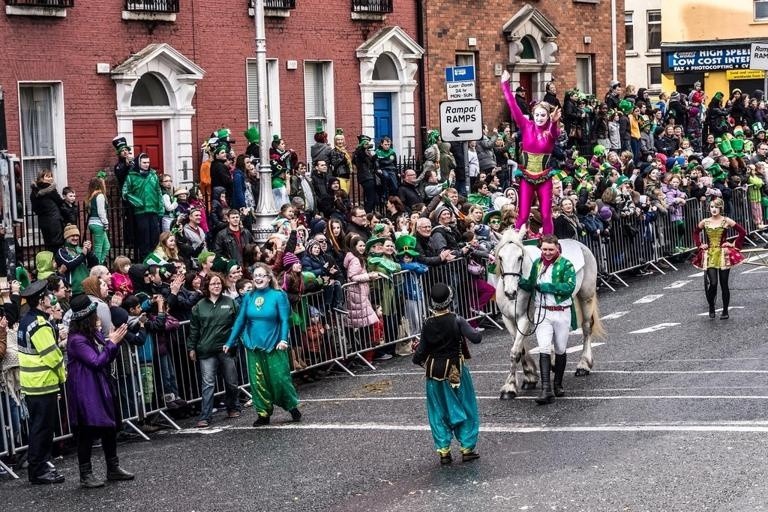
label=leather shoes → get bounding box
[437,447,452,464]
[229,409,241,418]
[709,307,716,319]
[720,313,729,319]
[462,449,481,461]
[28,475,64,484]
[47,467,59,472]
[197,419,208,427]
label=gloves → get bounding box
[534,284,540,294]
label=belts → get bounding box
[541,305,573,311]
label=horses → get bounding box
[495,223,607,402]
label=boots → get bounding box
[534,354,555,405]
[175,397,187,405]
[556,353,566,397]
[80,461,106,488]
[164,392,177,409]
[106,456,136,480]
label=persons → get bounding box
[487,175,503,193]
[311,208,326,234]
[416,219,457,298]
[187,273,240,428]
[396,234,428,335]
[61,187,78,227]
[159,174,177,235]
[310,160,331,200]
[365,235,401,362]
[408,212,419,225]
[445,187,458,205]
[146,231,178,264]
[302,239,349,315]
[322,178,350,218]
[111,136,137,262]
[172,185,194,225]
[111,256,132,297]
[150,297,187,411]
[223,263,301,428]
[460,220,497,332]
[110,306,147,443]
[15,280,68,486]
[147,265,162,293]
[342,236,379,367]
[515,86,534,121]
[87,177,110,266]
[351,134,384,214]
[424,169,456,199]
[211,186,230,250]
[551,81,767,291]
[270,159,287,192]
[66,294,135,488]
[55,224,100,297]
[493,132,518,188]
[268,136,298,176]
[366,211,380,232]
[412,189,447,218]
[215,210,258,273]
[196,250,215,281]
[395,212,410,238]
[500,69,561,238]
[313,233,344,288]
[399,169,423,215]
[122,294,165,434]
[383,237,395,261]
[288,163,317,213]
[543,82,561,110]
[499,125,510,138]
[81,276,115,339]
[504,187,518,210]
[128,263,185,309]
[499,122,510,129]
[248,164,260,207]
[121,153,165,262]
[518,234,577,406]
[35,250,66,279]
[328,128,351,180]
[290,197,307,229]
[375,223,391,238]
[411,283,483,465]
[483,211,501,247]
[48,294,63,324]
[375,137,399,199]
[324,219,348,283]
[271,204,294,236]
[386,196,404,232]
[46,275,67,303]
[231,154,256,210]
[422,145,441,181]
[159,264,178,296]
[427,130,441,145]
[467,181,493,206]
[90,266,112,306]
[439,142,457,180]
[428,206,468,260]
[29,168,70,256]
[476,166,498,186]
[294,224,308,254]
[311,132,332,166]
[0,280,22,327]
[467,140,479,194]
[346,206,371,242]
[693,197,747,320]
[189,186,210,233]
[466,205,483,226]
[498,204,515,233]
[475,121,498,174]
[209,147,232,191]
[183,207,205,252]
[276,252,307,371]
[244,127,260,165]
[169,213,206,271]
[0,315,7,362]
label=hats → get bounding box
[665,159,677,170]
[356,135,371,149]
[599,207,612,218]
[731,139,745,156]
[282,252,300,267]
[173,186,190,195]
[22,280,54,302]
[731,89,742,94]
[616,175,632,188]
[305,239,320,253]
[638,114,652,128]
[729,165,741,176]
[619,100,634,115]
[427,283,456,311]
[428,130,439,148]
[244,127,261,144]
[366,235,385,248]
[61,223,80,239]
[461,231,474,243]
[308,303,324,316]
[516,87,527,92]
[112,139,129,155]
[333,128,345,137]
[483,210,502,224]
[707,162,729,183]
[718,141,736,157]
[750,121,766,138]
[670,91,680,99]
[208,141,228,156]
[210,255,240,277]
[396,235,420,256]
[217,130,230,139]
[433,206,450,222]
[70,293,98,319]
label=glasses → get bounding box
[162,180,173,183]
[420,225,431,229]
[252,274,270,278]
[319,241,328,244]
[406,174,416,177]
[488,220,502,225]
[207,282,221,286]
[145,273,152,278]
[354,214,367,218]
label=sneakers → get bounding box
[252,416,270,426]
[139,424,160,433]
[475,327,485,331]
[295,348,307,368]
[470,307,486,316]
[289,352,302,369]
[375,354,393,360]
[289,407,302,421]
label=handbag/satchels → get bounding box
[395,316,415,358]
